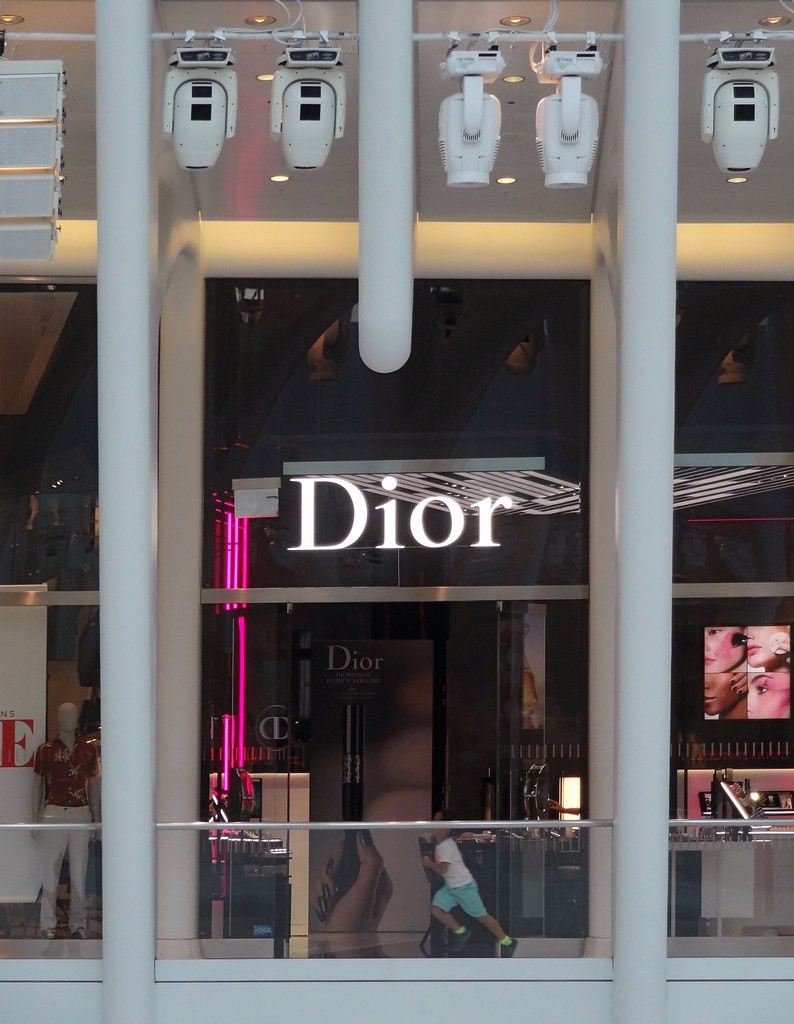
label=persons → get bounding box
[704,672,748,719]
[548,798,580,817]
[748,626,790,672]
[310,830,394,952]
[30,702,102,939]
[704,628,748,672]
[748,673,790,719]
[422,809,519,958]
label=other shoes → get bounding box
[452,929,472,952]
[501,938,518,958]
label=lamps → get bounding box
[162,48,238,171]
[438,50,507,187]
[702,48,780,175]
[268,48,347,172]
[534,51,605,188]
[0,59,65,263]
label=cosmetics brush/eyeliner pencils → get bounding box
[731,632,748,649]
[342,703,365,881]
